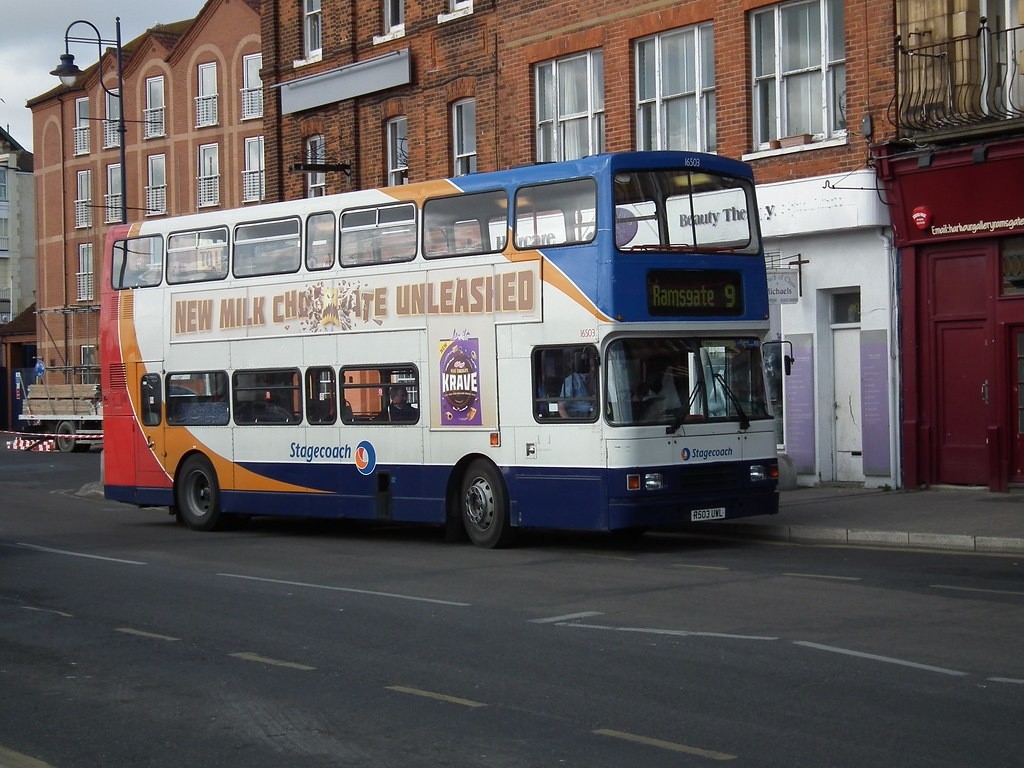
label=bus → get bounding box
[100,150,796,546]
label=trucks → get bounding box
[19,368,171,451]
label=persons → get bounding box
[557,346,599,418]
[373,385,418,421]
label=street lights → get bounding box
[49,17,162,244]
